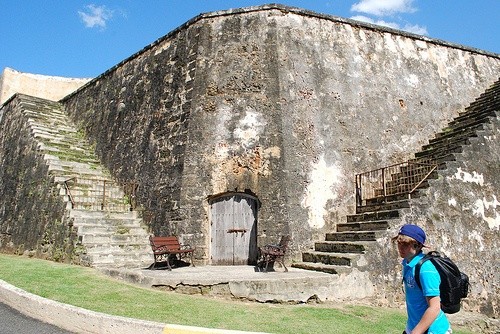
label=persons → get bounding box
[396,225,453,334]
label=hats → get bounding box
[399,225,426,247]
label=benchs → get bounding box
[149,234,197,272]
[259,236,290,273]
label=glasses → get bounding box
[401,279,404,293]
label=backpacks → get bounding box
[415,251,469,314]
